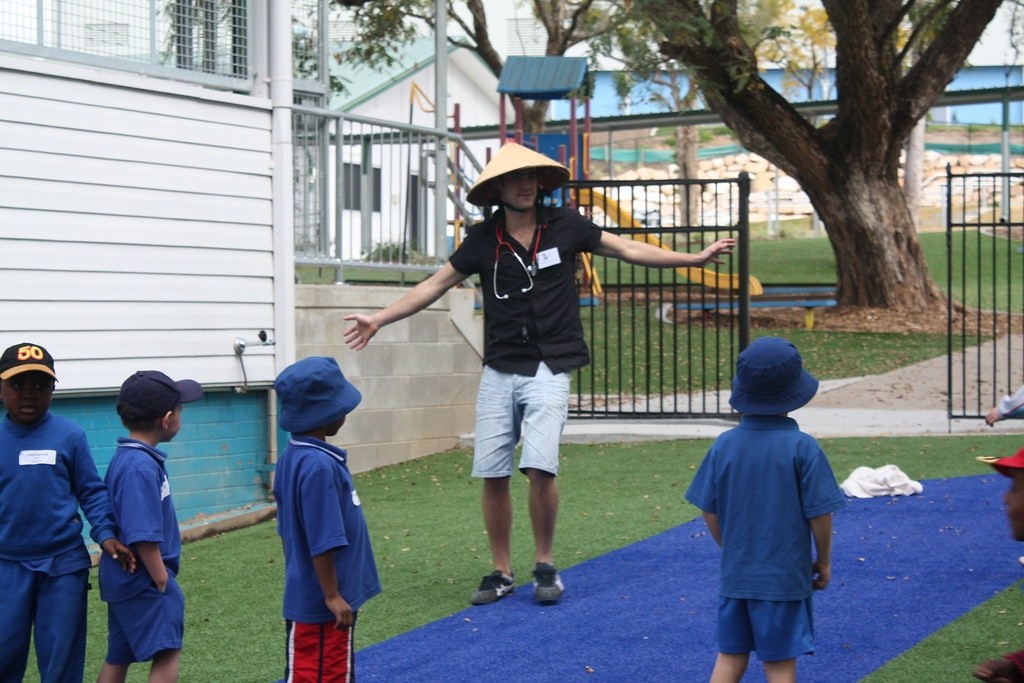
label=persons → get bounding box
[681,338,842,683]
[343,139,736,605]
[271,355,383,682]
[0,344,136,683]
[967,448,1024,683]
[987,384,1024,427]
[94,371,210,681]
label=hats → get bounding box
[466,142,570,206]
[728,336,818,415]
[0,343,59,383]
[116,370,204,421]
[273,356,362,433]
[991,447,1024,478]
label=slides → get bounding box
[578,188,763,295]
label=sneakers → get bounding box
[470,570,516,604]
[531,562,565,601]
